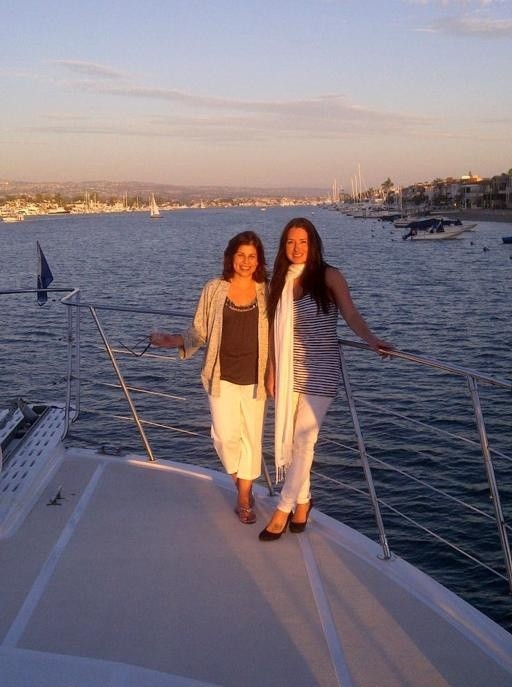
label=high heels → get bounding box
[258,509,294,542]
[288,496,315,534]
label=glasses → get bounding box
[118,335,152,358]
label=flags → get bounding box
[36,241,54,308]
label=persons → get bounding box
[258,218,397,542]
[151,231,271,525]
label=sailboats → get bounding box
[149,192,164,218]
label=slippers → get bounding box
[234,477,258,524]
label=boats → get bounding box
[320,162,477,242]
[1,286,511,685]
[502,235,511,244]
